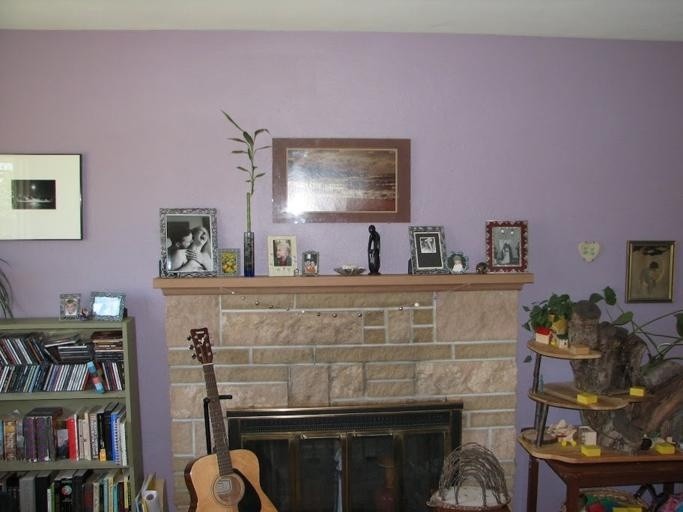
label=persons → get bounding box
[303,261,316,274]
[450,254,464,272]
[499,240,514,265]
[274,240,292,268]
[420,237,437,256]
[637,262,662,299]
[63,299,77,317]
[172,227,214,274]
[169,229,193,257]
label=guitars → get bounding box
[184,327,278,512]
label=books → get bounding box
[0,467,131,512]
[0,401,126,461]
[0,329,126,394]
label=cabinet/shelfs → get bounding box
[226,401,464,512]
[0,316,145,512]
[516,337,683,512]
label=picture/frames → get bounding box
[624,239,677,304]
[159,207,219,279]
[485,220,529,274]
[86,291,127,322]
[58,293,81,322]
[267,235,298,277]
[444,249,470,275]
[302,249,319,277]
[272,137,411,224]
[217,248,241,278]
[0,153,83,242]
[407,225,449,276]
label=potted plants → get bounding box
[219,107,272,277]
[521,285,634,333]
